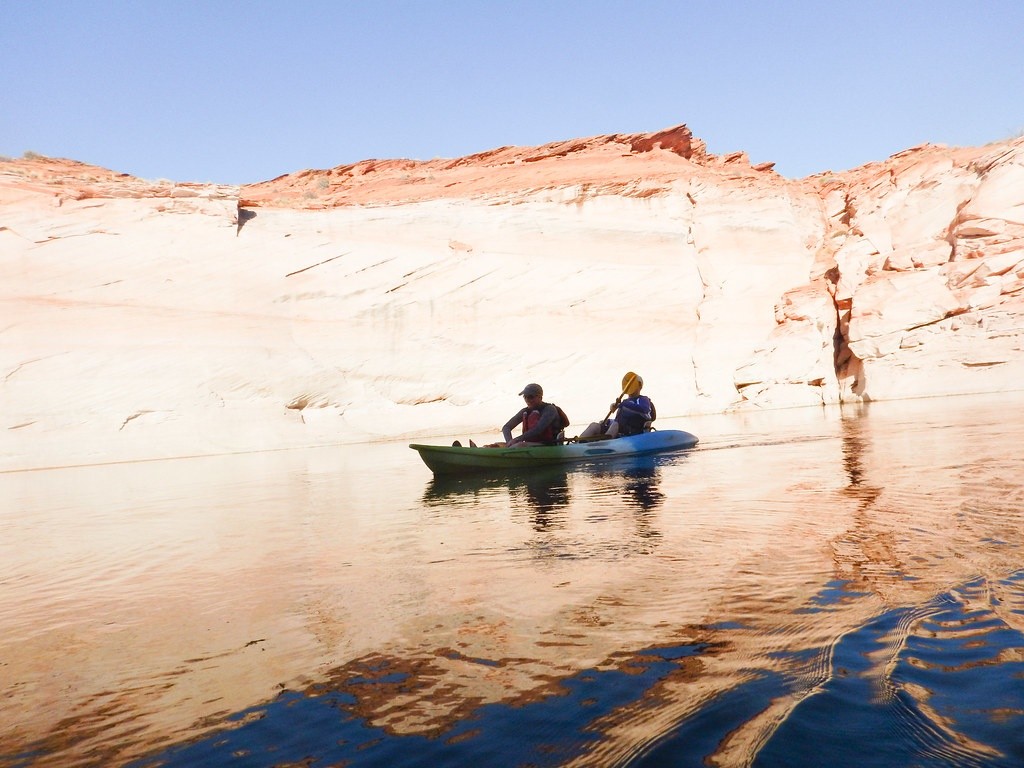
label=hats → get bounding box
[518,383,543,396]
[638,376,643,387]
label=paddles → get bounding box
[602,371,640,425]
[494,433,608,447]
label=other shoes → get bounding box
[452,441,462,447]
[469,439,477,448]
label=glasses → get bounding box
[524,395,535,399]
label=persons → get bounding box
[578,376,656,439]
[451,383,570,449]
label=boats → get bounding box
[408,429,699,477]
